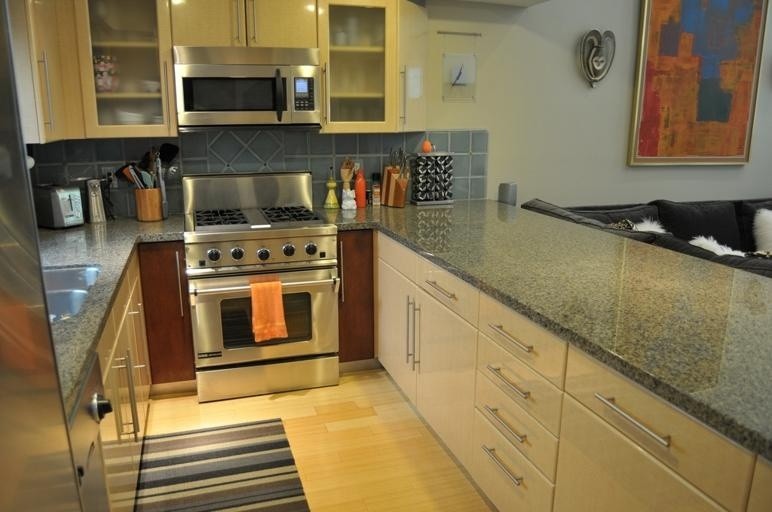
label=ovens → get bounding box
[185,258,341,404]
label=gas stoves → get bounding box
[183,204,339,277]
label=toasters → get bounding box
[33,181,86,229]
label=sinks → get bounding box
[43,262,100,289]
[41,289,88,324]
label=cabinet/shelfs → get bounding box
[171,0,317,48]
[372,201,772,512]
[74,0,177,138]
[6,0,86,144]
[318,0,427,133]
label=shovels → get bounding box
[159,143,179,167]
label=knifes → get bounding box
[388,146,409,180]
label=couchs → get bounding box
[521,197,772,278]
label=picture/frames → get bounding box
[626,0,769,167]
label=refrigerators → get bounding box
[2,1,90,512]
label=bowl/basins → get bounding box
[114,78,163,125]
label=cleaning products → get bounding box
[354,169,367,208]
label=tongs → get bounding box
[154,156,171,219]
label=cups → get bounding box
[333,14,360,47]
[135,188,163,222]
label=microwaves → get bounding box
[171,44,323,134]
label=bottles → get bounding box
[87,180,107,224]
[323,166,341,210]
[421,132,432,153]
[354,168,382,208]
